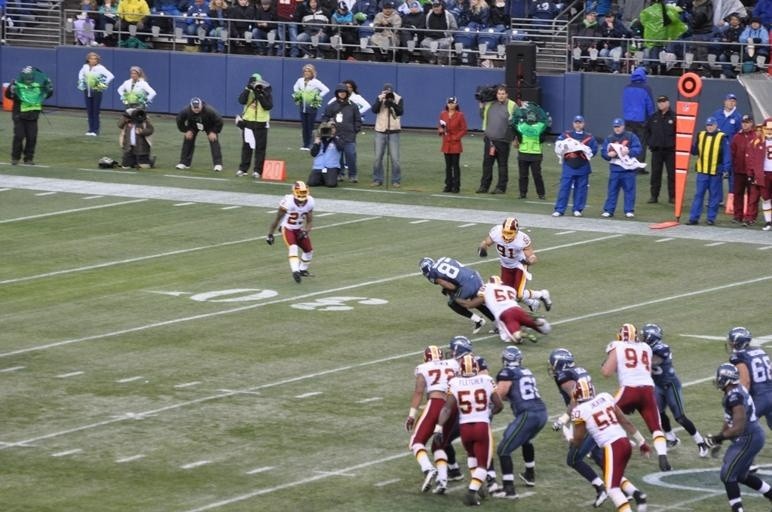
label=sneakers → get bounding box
[443,185,459,193]
[601,212,634,218]
[593,482,648,512]
[12,159,34,165]
[646,196,657,203]
[293,270,315,283]
[667,437,679,452]
[472,318,498,334]
[749,463,758,473]
[520,290,552,342]
[551,210,582,216]
[176,163,190,170]
[336,173,358,183]
[697,434,722,457]
[391,181,400,188]
[520,190,545,199]
[212,164,223,171]
[476,186,505,194]
[660,455,672,471]
[236,169,261,179]
[85,132,96,136]
[369,180,383,187]
[669,197,675,203]
[422,464,535,505]
[684,200,772,231]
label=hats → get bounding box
[20,65,34,78]
[572,115,584,122]
[433,0,440,7]
[336,1,348,10]
[741,114,752,122]
[446,96,458,104]
[612,117,624,127]
[190,96,202,108]
[383,82,393,91]
[705,116,717,125]
[724,93,736,100]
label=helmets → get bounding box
[292,180,310,202]
[488,275,502,285]
[763,119,772,138]
[548,349,575,376]
[418,258,434,277]
[502,217,518,242]
[639,323,662,346]
[712,363,739,389]
[353,12,367,20]
[573,380,595,402]
[501,346,522,367]
[725,326,752,354]
[616,323,639,342]
[424,336,479,377]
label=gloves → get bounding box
[641,441,651,457]
[519,257,529,264]
[433,432,442,445]
[477,247,488,258]
[552,418,574,441]
[297,231,307,241]
[405,416,415,431]
[267,234,274,245]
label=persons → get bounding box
[400,322,772,512]
[115,106,156,169]
[117,65,158,110]
[417,255,500,335]
[265,180,316,285]
[174,96,225,171]
[476,217,552,312]
[233,72,275,179]
[0,0,771,83]
[448,275,551,345]
[76,51,115,137]
[3,64,54,166]
[288,63,771,231]
